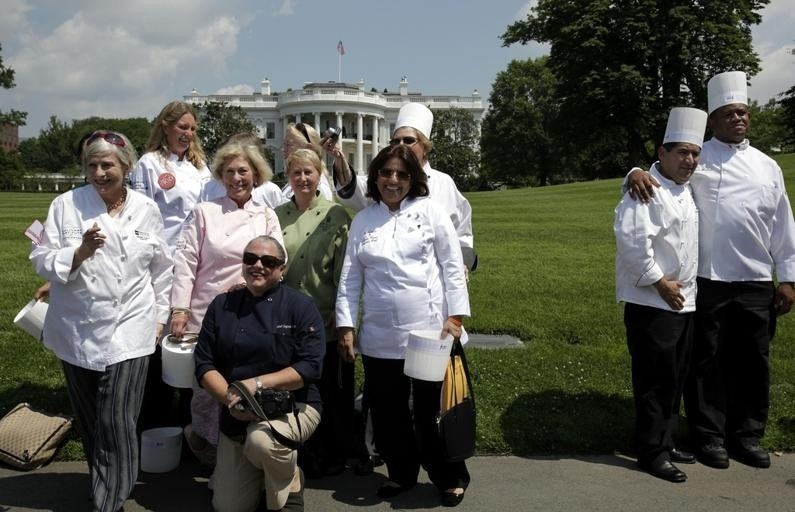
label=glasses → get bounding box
[87,132,126,151]
[243,251,285,267]
[295,121,311,143]
[167,333,199,344]
[378,167,412,179]
[389,136,417,145]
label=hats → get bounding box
[393,103,434,138]
[708,70,749,113]
[13,299,49,344]
[663,105,708,148]
[141,428,182,474]
[161,333,199,388]
[403,331,454,380]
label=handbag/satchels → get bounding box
[438,336,475,463]
[355,382,383,466]
[219,381,303,449]
[0,403,73,471]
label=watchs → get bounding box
[252,374,264,395]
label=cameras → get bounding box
[320,125,341,152]
[234,386,293,421]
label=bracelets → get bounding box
[171,310,189,316]
[449,317,463,327]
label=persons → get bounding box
[199,132,284,210]
[620,72,794,468]
[333,102,479,284]
[282,122,334,204]
[338,145,473,506]
[29,131,175,512]
[170,144,288,342]
[274,149,352,475]
[133,103,214,428]
[615,107,708,482]
[195,236,324,511]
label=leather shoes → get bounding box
[325,461,344,475]
[380,483,417,498]
[439,490,463,506]
[354,464,373,477]
[695,444,728,468]
[731,445,771,469]
[671,449,695,464]
[257,491,269,511]
[283,468,305,512]
[637,458,686,484]
